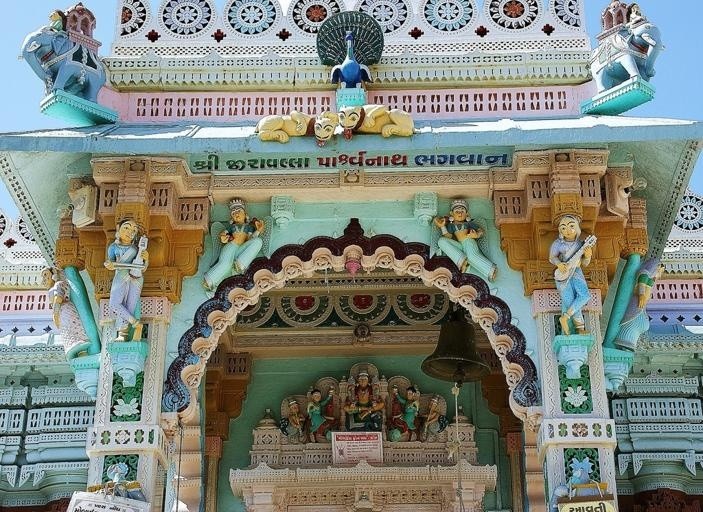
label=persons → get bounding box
[202,197,264,290]
[613,258,662,352]
[549,215,592,335]
[105,219,149,342]
[434,200,497,282]
[280,372,450,442]
[41,267,90,360]
